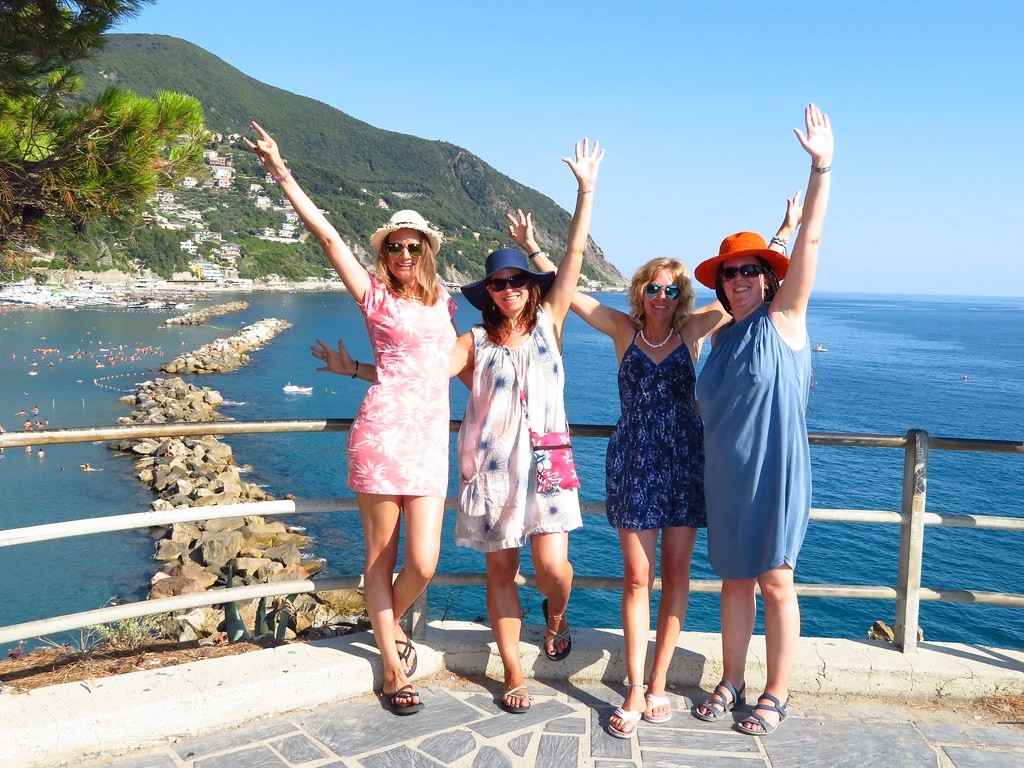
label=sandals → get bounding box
[695,680,744,721]
[738,693,789,735]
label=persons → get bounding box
[695,103,834,736]
[243,121,474,715]
[505,191,804,737]
[311,137,604,714]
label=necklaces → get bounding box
[397,287,421,299]
[640,327,674,347]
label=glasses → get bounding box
[489,274,529,292]
[383,242,424,257]
[719,264,767,280]
[641,282,681,301]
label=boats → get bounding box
[812,343,828,352]
[283,382,313,394]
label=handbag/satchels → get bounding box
[530,431,581,492]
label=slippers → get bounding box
[542,598,572,661]
[643,694,672,724]
[608,707,642,739]
[384,684,425,714]
[500,684,531,712]
[395,636,418,678]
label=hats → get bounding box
[461,248,555,312]
[694,231,790,289]
[369,209,442,257]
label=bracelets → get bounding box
[352,360,359,379]
[271,169,291,180]
[577,190,594,194]
[811,166,832,173]
[771,236,788,259]
[528,249,544,259]
